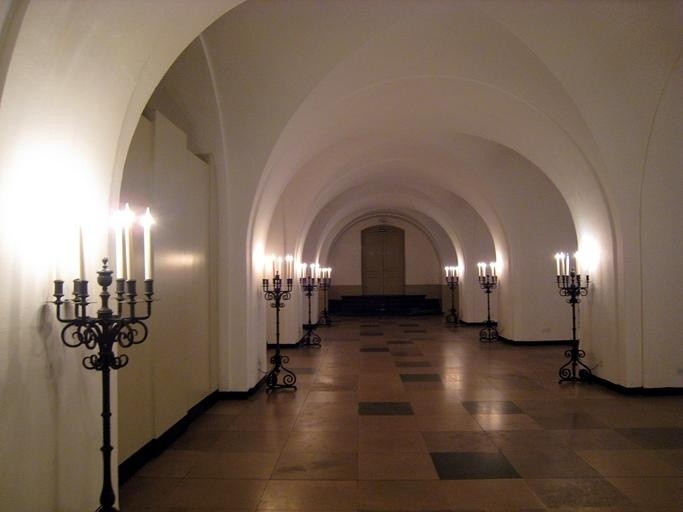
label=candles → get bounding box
[555,250,590,275]
[446,267,458,277]
[478,263,497,276]
[262,253,332,280]
[50,202,155,283]
